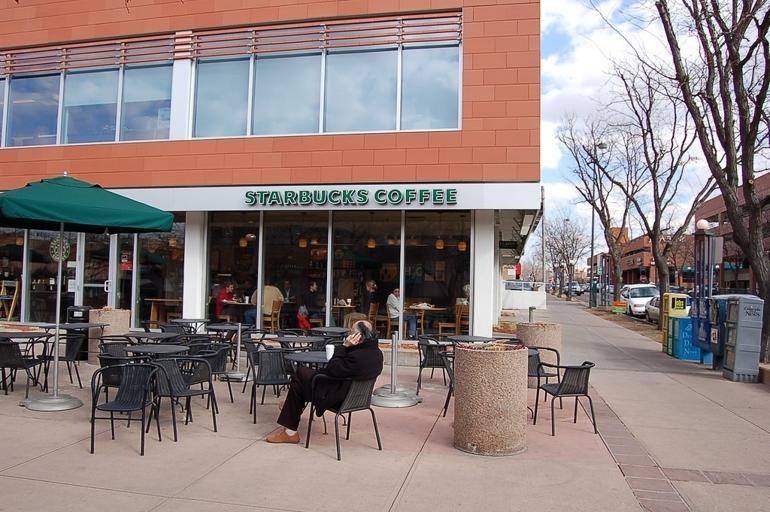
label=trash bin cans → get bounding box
[65,306,93,361]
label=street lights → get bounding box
[563,218,572,254]
[715,264,720,284]
[689,219,719,326]
[687,266,691,288]
[597,246,614,309]
[589,139,611,310]
[551,258,573,299]
[532,253,538,282]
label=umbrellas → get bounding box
[0,171,175,396]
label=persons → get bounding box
[266,320,383,443]
[462,283,470,305]
[210,279,417,340]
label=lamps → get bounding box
[435,211,445,250]
[367,211,377,249]
[457,215,467,252]
[298,212,307,247]
[239,211,247,247]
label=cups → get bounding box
[325,344,334,361]
[244,296,249,304]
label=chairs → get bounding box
[526,345,562,409]
[494,337,521,345]
[533,361,597,436]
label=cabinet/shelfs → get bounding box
[264,254,365,312]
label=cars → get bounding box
[543,280,618,296]
[618,281,723,330]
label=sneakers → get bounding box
[266,427,300,443]
[279,399,286,410]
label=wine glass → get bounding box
[346,299,351,307]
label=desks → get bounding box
[446,334,496,397]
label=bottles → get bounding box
[308,260,325,269]
[408,264,424,276]
[32,279,45,289]
[333,255,363,281]
